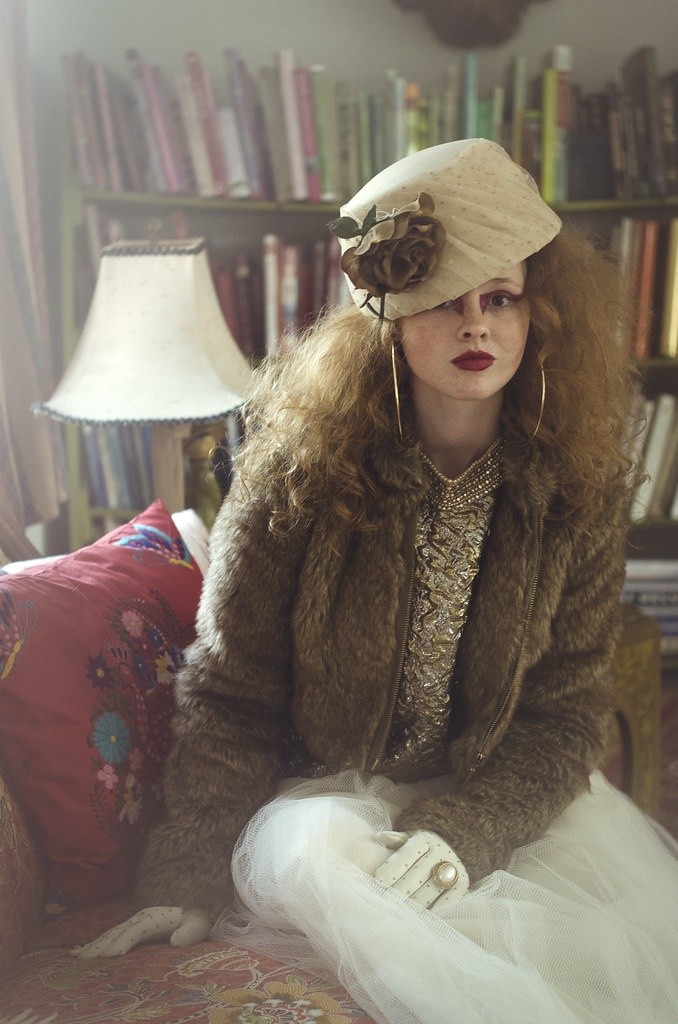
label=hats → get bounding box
[323,140,563,324]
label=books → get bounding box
[74,45,678,533]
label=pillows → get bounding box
[0,499,205,927]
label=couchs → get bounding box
[0,508,380,1023]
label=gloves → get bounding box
[372,826,471,920]
[71,905,216,958]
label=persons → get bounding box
[72,136,678,1024]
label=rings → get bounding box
[432,860,458,889]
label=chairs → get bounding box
[182,435,662,823]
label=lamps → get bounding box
[31,218,257,515]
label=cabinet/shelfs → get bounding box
[53,70,678,663]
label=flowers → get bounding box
[325,193,447,323]
